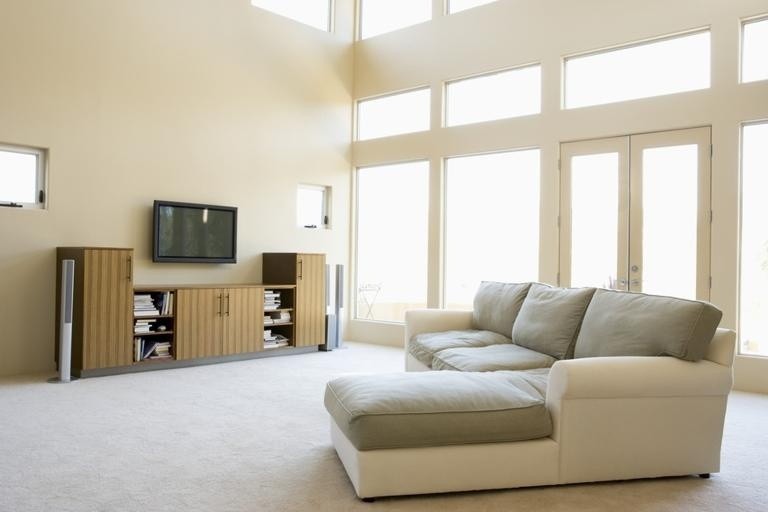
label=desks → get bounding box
[55,247,325,378]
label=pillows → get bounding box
[573,288,723,360]
[473,280,531,339]
[512,283,596,358]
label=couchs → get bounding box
[325,280,737,502]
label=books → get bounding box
[265,291,291,348]
[133,292,174,362]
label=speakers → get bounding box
[47,260,79,382]
[336,264,344,349]
[320,315,336,351]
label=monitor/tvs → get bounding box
[153,200,237,264]
